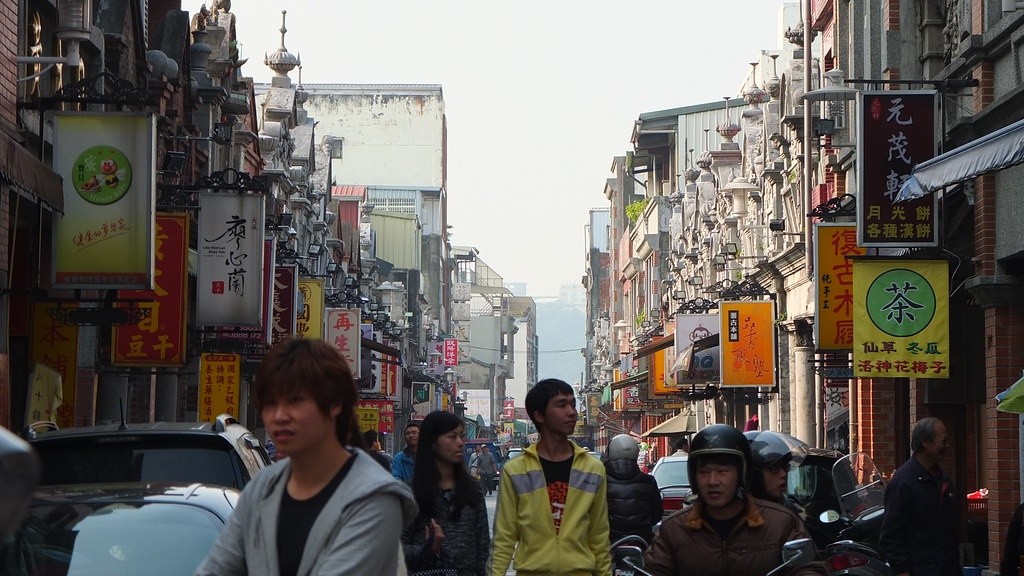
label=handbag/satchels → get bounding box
[408,535,458,576]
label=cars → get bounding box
[647,453,697,523]
[461,439,502,491]
[1,479,253,576]
[17,414,276,497]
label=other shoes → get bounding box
[489,490,492,495]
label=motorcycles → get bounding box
[604,534,819,576]
[809,450,907,575]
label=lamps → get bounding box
[641,217,802,329]
[162,116,403,339]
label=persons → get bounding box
[878,418,965,576]
[346,410,809,547]
[486,379,612,576]
[399,410,489,576]
[635,424,826,576]
[191,339,419,576]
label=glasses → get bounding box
[773,463,790,473]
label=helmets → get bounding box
[742,430,809,481]
[687,424,752,498]
[606,434,639,461]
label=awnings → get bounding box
[892,119,1024,205]
[638,411,696,437]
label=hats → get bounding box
[481,444,486,448]
